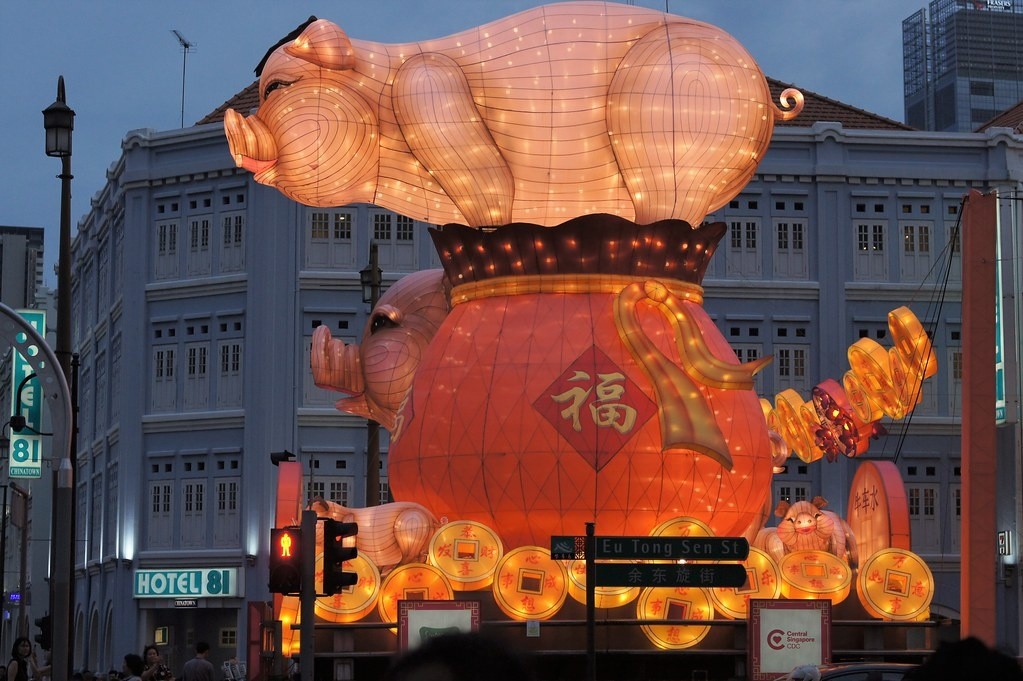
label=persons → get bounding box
[789,635,1023,681]
[0,638,216,681]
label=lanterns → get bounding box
[221,4,937,651]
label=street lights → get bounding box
[41,76,72,681]
